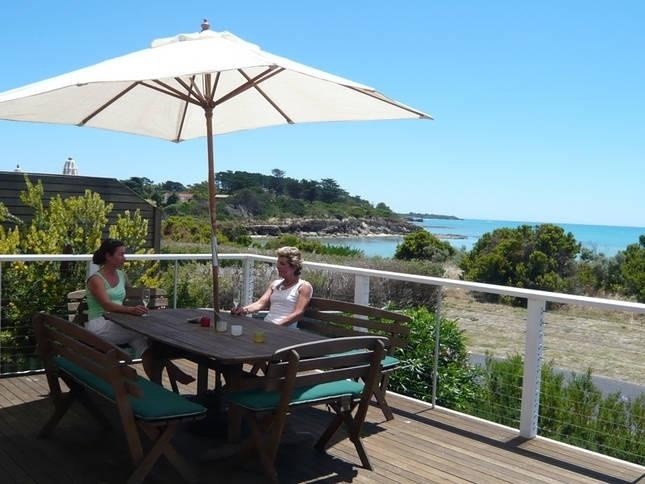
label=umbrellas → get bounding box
[0,17,434,397]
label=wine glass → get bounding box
[233,290,240,311]
[142,288,151,317]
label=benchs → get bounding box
[258,298,413,442]
[31,311,208,484]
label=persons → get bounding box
[219,245,314,384]
[86,238,195,388]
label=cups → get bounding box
[200,311,265,344]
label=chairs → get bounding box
[68,289,181,394]
[230,336,387,484]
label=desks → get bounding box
[102,308,333,464]
